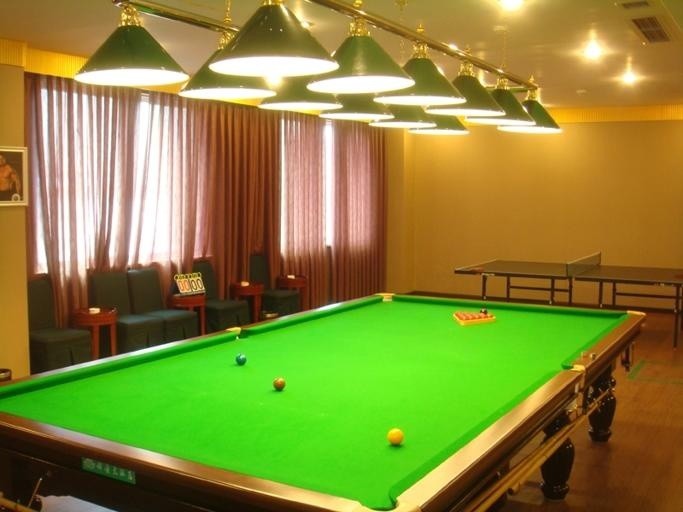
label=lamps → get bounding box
[206,1,340,79]
[421,40,505,118]
[73,0,190,89]
[257,75,469,135]
[371,22,468,108]
[176,0,277,104]
[304,1,415,96]
[496,73,561,135]
[467,60,535,129]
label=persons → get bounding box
[0,154,21,201]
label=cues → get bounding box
[461,390,612,511]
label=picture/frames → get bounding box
[0,146,28,207]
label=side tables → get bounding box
[275,277,310,310]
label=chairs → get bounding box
[28,255,299,376]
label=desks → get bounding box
[456,255,683,350]
[0,292,647,512]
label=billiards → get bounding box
[454,309,493,320]
[273,378,284,390]
[388,428,402,444]
[236,355,246,365]
